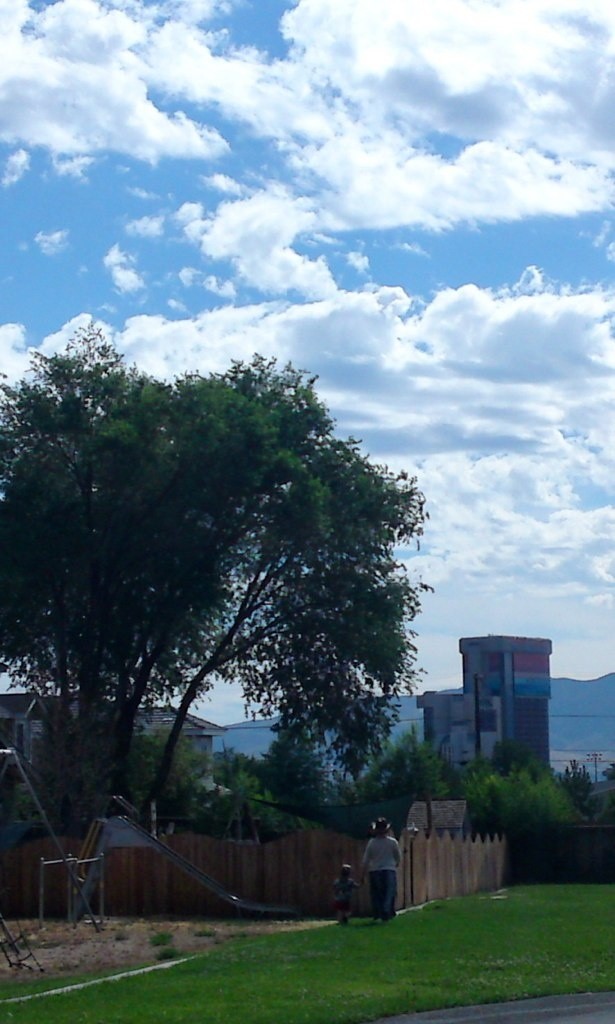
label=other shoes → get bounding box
[380,911,389,922]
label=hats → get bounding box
[372,817,391,834]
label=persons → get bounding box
[328,864,360,924]
[358,818,401,922]
